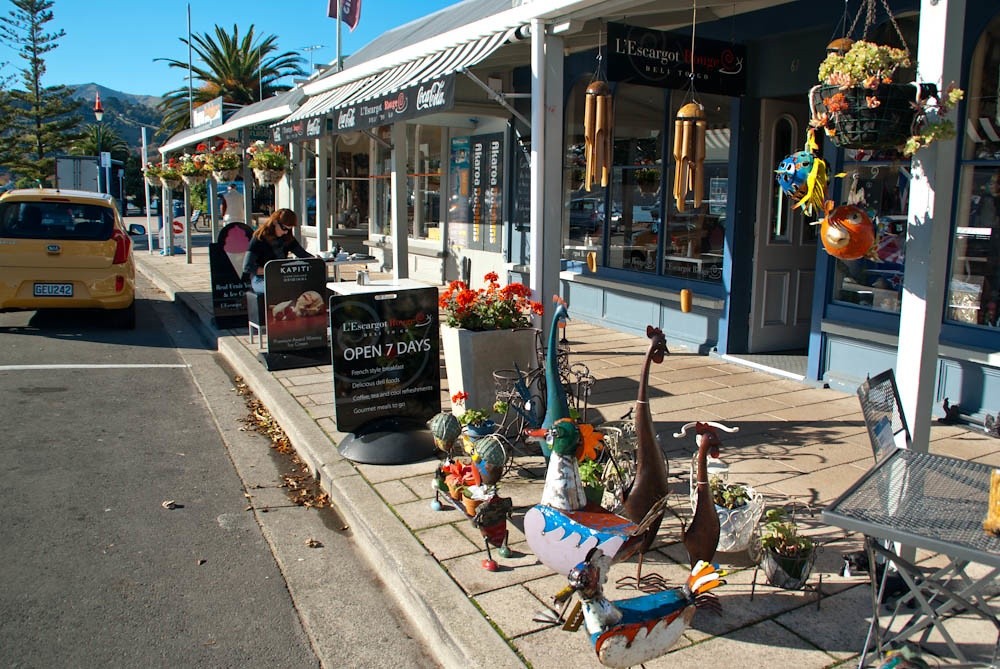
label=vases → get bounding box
[441,321,544,437]
[813,82,930,149]
[182,175,205,185]
[147,176,162,186]
[160,177,180,189]
[212,169,238,182]
[254,168,285,186]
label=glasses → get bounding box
[277,220,291,232]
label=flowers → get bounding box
[820,42,965,155]
[178,155,207,176]
[141,162,162,177]
[195,143,238,172]
[156,159,181,181]
[247,145,291,171]
[439,273,545,331]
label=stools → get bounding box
[245,292,266,349]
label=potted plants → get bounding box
[579,458,607,507]
[705,478,767,552]
[756,501,819,590]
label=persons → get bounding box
[241,208,316,294]
[220,183,244,226]
[967,172,1000,275]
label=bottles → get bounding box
[583,236,593,246]
[356,270,369,285]
[681,288,691,314]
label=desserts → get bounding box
[272,290,324,320]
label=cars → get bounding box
[0,190,137,320]
[568,199,623,232]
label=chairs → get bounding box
[858,367,992,608]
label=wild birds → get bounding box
[523,294,740,669]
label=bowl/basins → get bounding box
[338,254,347,260]
[319,251,331,258]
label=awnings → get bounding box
[332,26,523,136]
[268,73,376,146]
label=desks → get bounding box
[565,242,725,280]
[821,446,1000,669]
[324,276,434,295]
[324,257,379,283]
